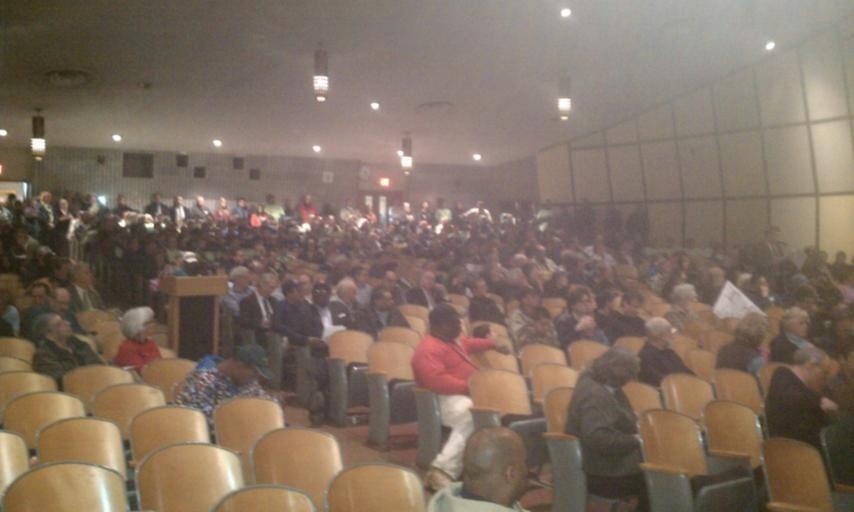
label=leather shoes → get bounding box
[422,467,453,492]
[309,390,325,424]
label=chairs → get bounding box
[0,269,853,510]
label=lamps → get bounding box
[28,104,49,165]
[398,129,416,177]
[310,38,331,105]
[555,69,572,122]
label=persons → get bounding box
[1,189,852,493]
[427,424,533,512]
[563,348,649,511]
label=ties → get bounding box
[263,300,271,319]
[82,292,90,310]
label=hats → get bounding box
[281,279,305,294]
[429,301,466,327]
[235,342,275,380]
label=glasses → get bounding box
[669,327,679,336]
[312,289,328,296]
